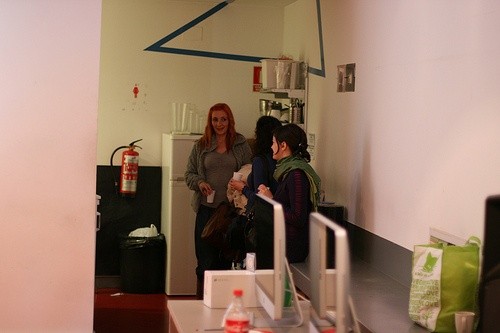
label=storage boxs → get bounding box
[203,270,262,310]
[255,269,274,300]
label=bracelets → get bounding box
[241,184,248,194]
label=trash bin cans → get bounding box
[118,232,165,294]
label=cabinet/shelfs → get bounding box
[260,77,308,132]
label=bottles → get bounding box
[223,288,249,333]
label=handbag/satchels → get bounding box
[409,235,482,332]
[201,202,234,243]
[221,213,253,263]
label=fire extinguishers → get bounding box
[110,139,143,199]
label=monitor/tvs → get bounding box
[252,193,360,333]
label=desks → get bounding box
[289,221,436,333]
[167,299,353,333]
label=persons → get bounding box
[184,103,252,301]
[258,123,315,264]
[230,115,282,271]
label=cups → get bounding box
[290,61,306,89]
[455,312,476,333]
[207,190,216,203]
[233,172,242,181]
[259,99,269,117]
